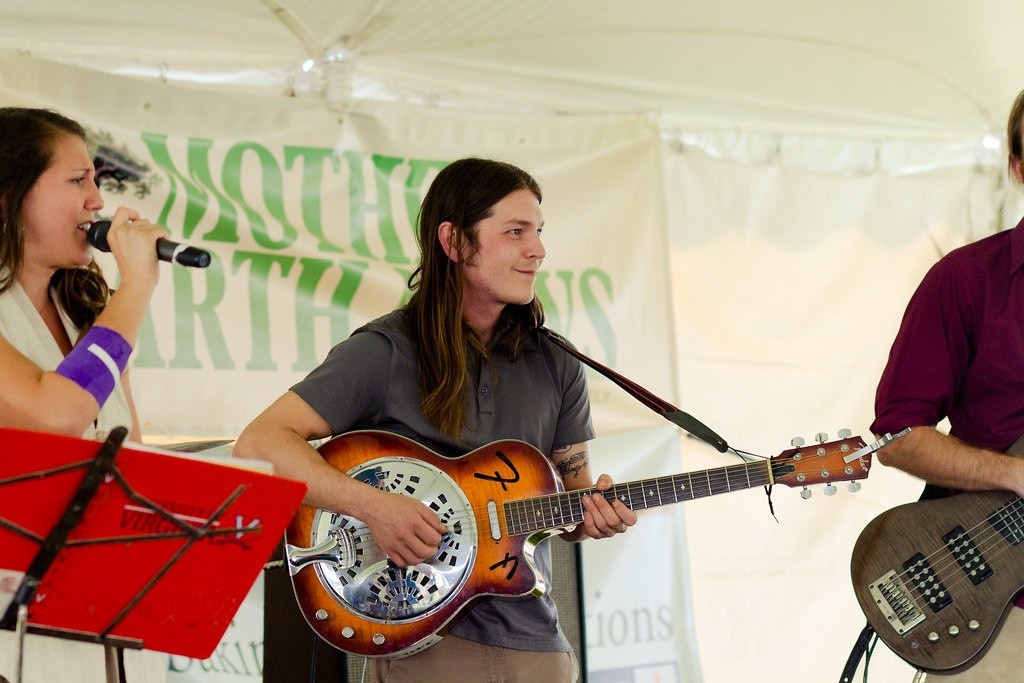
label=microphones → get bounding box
[87,220,211,269]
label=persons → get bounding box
[232,158,637,683]
[0,107,168,683]
[870,89,1024,683]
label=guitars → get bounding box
[849,433,1024,678]
[283,427,873,663]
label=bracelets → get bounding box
[54,325,132,410]
